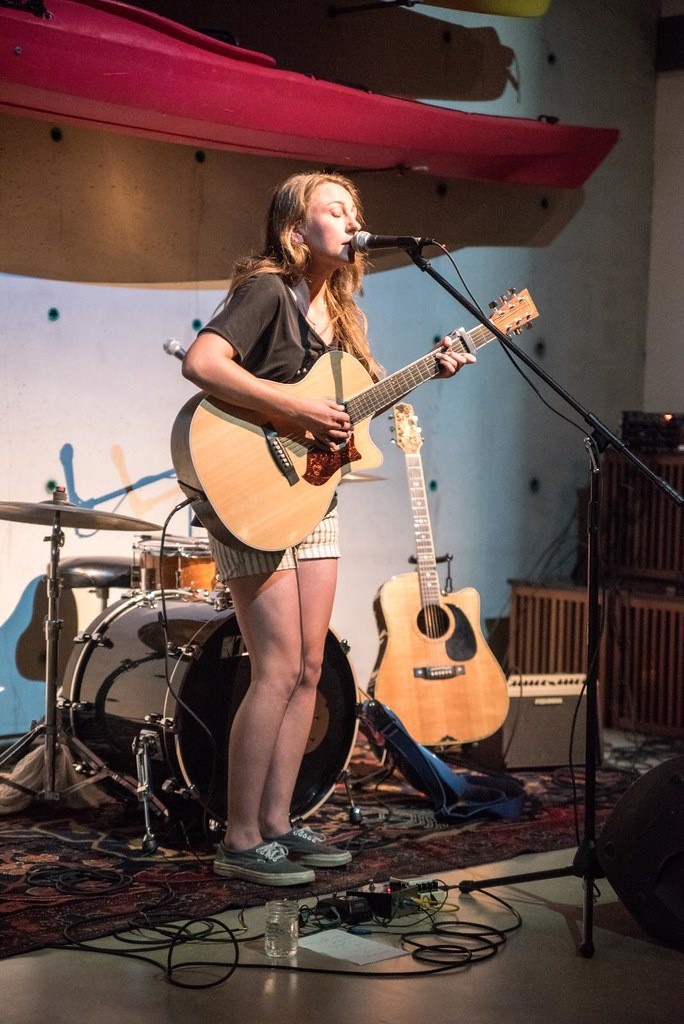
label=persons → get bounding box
[182,170,477,887]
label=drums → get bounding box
[132,540,230,597]
[58,589,362,831]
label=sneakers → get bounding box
[212,838,316,886]
[263,822,353,867]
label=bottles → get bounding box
[262,900,299,958]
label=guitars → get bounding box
[365,400,513,750]
[167,284,540,558]
[360,699,466,803]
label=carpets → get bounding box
[0,744,642,959]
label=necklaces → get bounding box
[306,289,327,325]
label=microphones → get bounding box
[163,339,186,360]
[350,230,436,255]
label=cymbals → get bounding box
[342,473,386,483]
[1,499,165,533]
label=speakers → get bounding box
[599,755,684,952]
[470,674,603,771]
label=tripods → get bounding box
[0,512,173,827]
[396,238,684,957]
